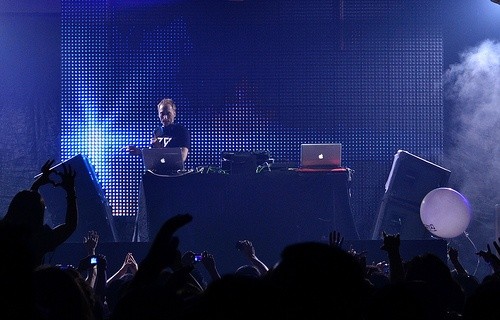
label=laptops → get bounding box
[300,144,341,169]
[140,148,184,175]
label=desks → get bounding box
[138,164,360,242]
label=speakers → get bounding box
[372,149,452,242]
[34,154,119,243]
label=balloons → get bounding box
[420,187,471,239]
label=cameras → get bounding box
[194,255,203,262]
[88,257,98,265]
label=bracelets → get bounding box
[88,254,97,258]
[63,196,78,200]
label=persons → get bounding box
[0,158,500,320]
[130,97,191,169]
